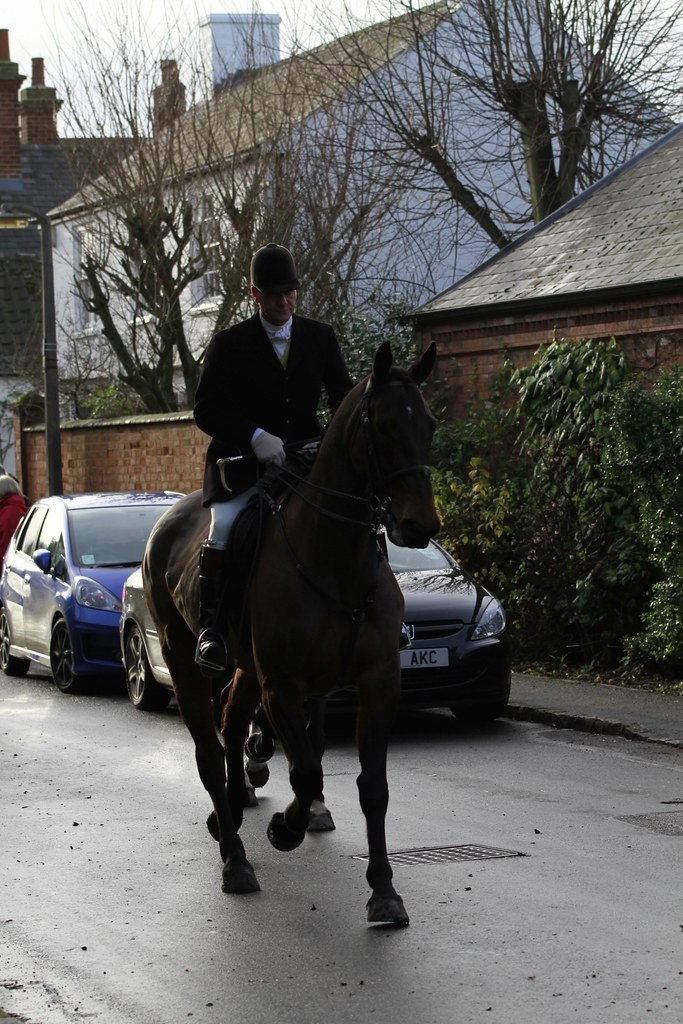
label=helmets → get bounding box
[251,243,300,295]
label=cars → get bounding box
[0,488,187,695]
[117,507,514,728]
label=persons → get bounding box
[0,465,32,578]
[191,243,353,670]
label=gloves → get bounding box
[252,431,286,468]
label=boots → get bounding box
[197,546,226,667]
[377,531,410,649]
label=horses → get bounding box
[140,339,441,926]
